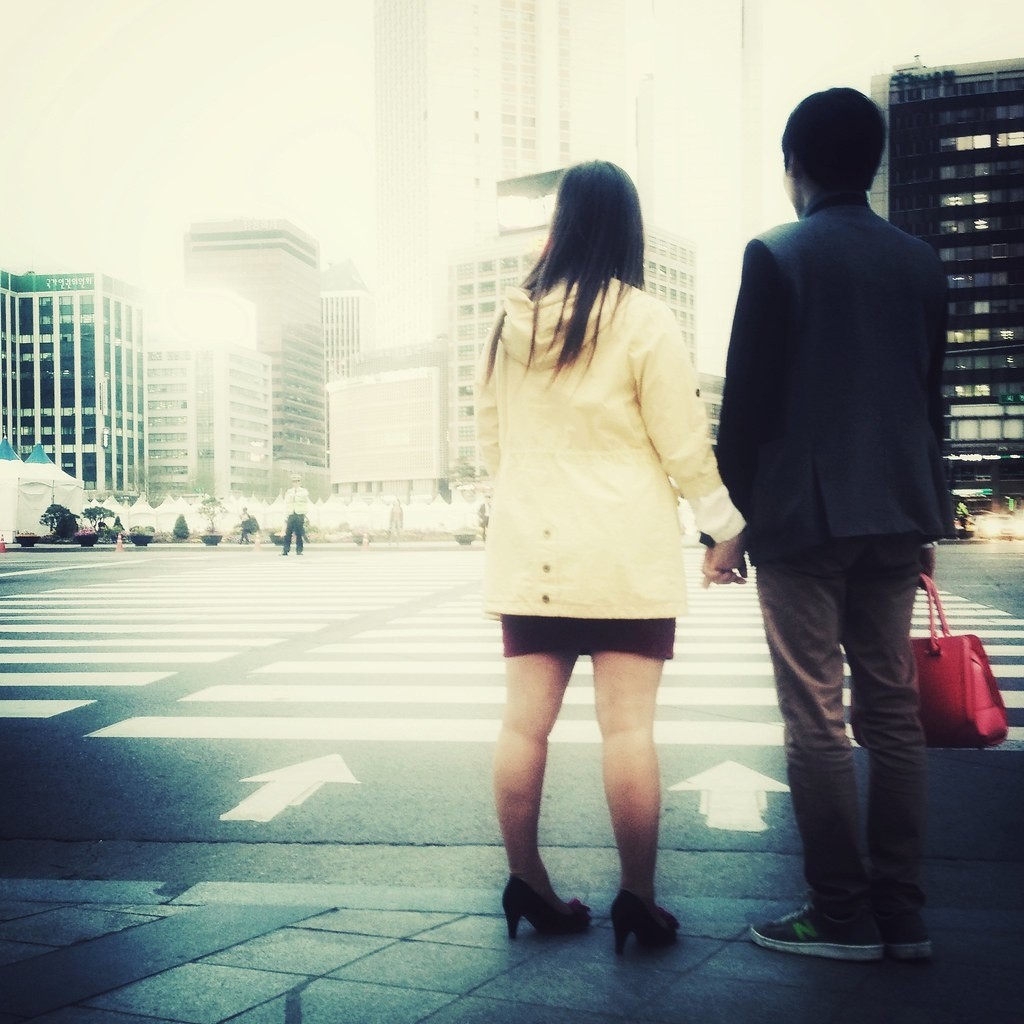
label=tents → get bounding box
[0,438,85,542]
[306,484,478,534]
[85,494,284,532]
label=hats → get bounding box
[291,474,301,481]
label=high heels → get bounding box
[502,873,591,940]
[611,889,679,955]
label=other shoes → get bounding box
[282,553,288,555]
[297,552,303,555]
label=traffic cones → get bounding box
[363,533,370,546]
[1,533,8,553]
[115,533,125,552]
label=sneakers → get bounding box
[748,895,885,961]
[871,907,932,961]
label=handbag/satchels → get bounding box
[911,572,1008,747]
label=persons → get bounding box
[279,474,311,556]
[957,498,973,530]
[238,507,252,544]
[477,495,492,544]
[389,498,404,546]
[700,90,958,964]
[472,161,748,951]
